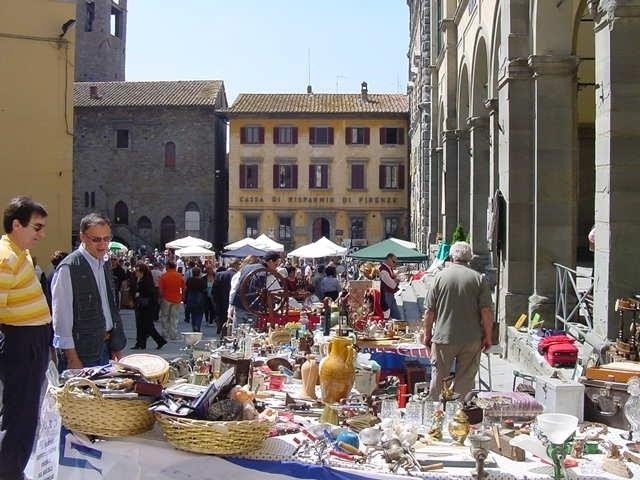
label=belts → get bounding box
[105,332,114,342]
[5,324,54,329]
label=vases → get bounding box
[318,338,356,403]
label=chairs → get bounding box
[512,369,536,397]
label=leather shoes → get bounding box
[156,341,168,350]
[131,343,145,349]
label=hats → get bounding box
[263,250,279,259]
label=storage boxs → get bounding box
[585,368,639,383]
[582,379,631,432]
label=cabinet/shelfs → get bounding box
[535,375,584,424]
[615,297,640,362]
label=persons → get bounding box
[0,197,53,480]
[281,253,376,305]
[230,250,280,329]
[211,261,239,325]
[157,260,186,340]
[185,268,208,332]
[128,247,217,281]
[131,263,167,350]
[52,212,127,379]
[378,253,401,322]
[40,250,67,316]
[422,241,493,404]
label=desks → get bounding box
[255,314,320,332]
[358,342,429,358]
[53,391,640,480]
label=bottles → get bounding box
[339,304,348,336]
[320,297,331,336]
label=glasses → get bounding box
[28,222,48,233]
[110,257,117,260]
[134,268,144,274]
[390,257,397,266]
[84,232,113,244]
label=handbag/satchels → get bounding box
[142,298,160,320]
[380,287,388,311]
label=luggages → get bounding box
[540,332,577,369]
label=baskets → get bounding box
[57,371,170,439]
[142,372,170,389]
[153,414,271,456]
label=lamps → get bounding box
[59,18,77,39]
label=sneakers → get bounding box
[172,336,182,338]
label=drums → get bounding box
[119,354,171,389]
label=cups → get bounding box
[445,401,463,418]
[424,401,444,424]
[404,403,423,425]
[381,399,402,421]
